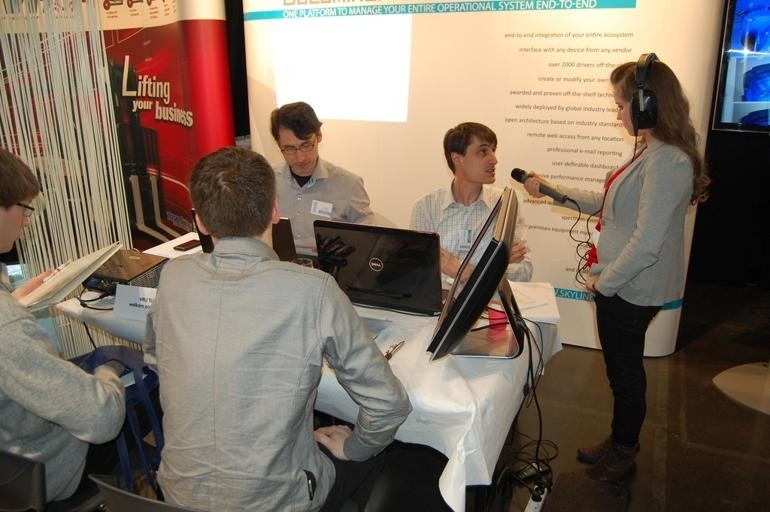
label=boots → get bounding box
[585,442,640,483]
[578,435,613,463]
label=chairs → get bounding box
[88,472,197,512]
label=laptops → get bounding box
[313,219,449,316]
[192,208,319,268]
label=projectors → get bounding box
[82,250,169,295]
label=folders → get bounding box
[18,240,125,313]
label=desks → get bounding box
[55,226,561,512]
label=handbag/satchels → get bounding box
[67,344,162,476]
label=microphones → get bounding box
[512,168,568,204]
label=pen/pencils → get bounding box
[381,340,406,361]
[42,259,71,283]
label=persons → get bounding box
[268,102,397,256]
[0,146,128,504]
[143,144,414,512]
[414,121,535,281]
[523,60,713,483]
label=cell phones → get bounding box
[173,240,201,251]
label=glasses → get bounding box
[16,202,35,217]
[278,135,318,154]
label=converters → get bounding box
[518,460,550,480]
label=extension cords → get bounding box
[524,486,547,512]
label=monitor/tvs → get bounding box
[426,186,525,362]
[708,0,770,134]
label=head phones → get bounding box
[630,53,656,128]
